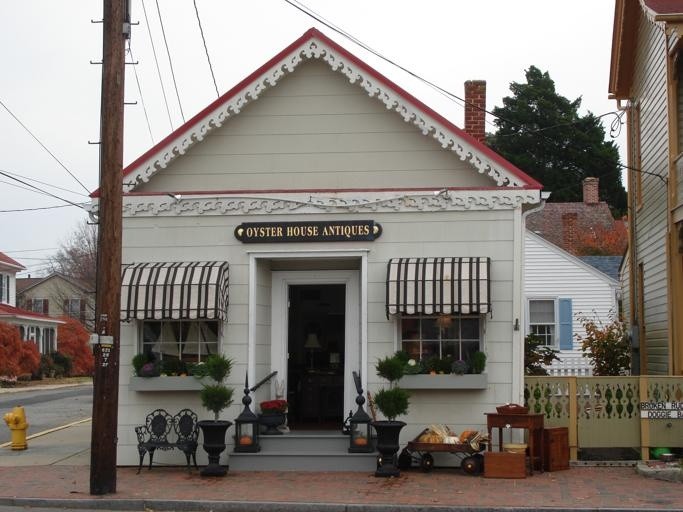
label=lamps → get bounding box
[304,333,322,373]
[348,370,373,453]
[233,372,261,452]
[89,312,114,369]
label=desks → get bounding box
[483,413,545,479]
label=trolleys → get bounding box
[398,426,481,474]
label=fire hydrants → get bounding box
[3,405,30,450]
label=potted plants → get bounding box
[370,356,410,477]
[197,356,234,476]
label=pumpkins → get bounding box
[419,425,473,445]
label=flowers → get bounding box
[260,399,288,415]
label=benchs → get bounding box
[135,408,200,475]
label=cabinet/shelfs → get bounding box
[531,426,569,471]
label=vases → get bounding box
[259,413,285,435]
